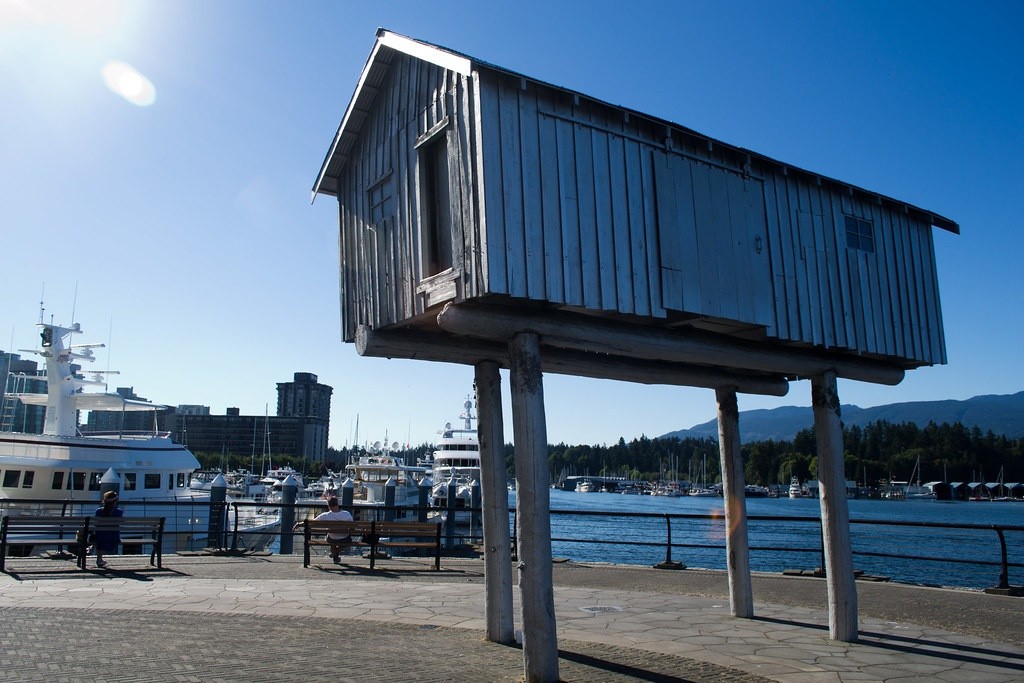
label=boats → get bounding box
[1,284,281,556]
[876,454,937,502]
[191,394,480,527]
[967,496,1024,501]
[789,476,802,498]
[551,452,769,497]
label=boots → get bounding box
[86,545,94,554]
[95,555,107,565]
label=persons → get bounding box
[292,498,353,564]
[86,492,123,567]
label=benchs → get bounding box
[303,519,442,574]
[0,515,165,572]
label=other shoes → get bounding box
[329,553,333,558]
[333,557,341,564]
